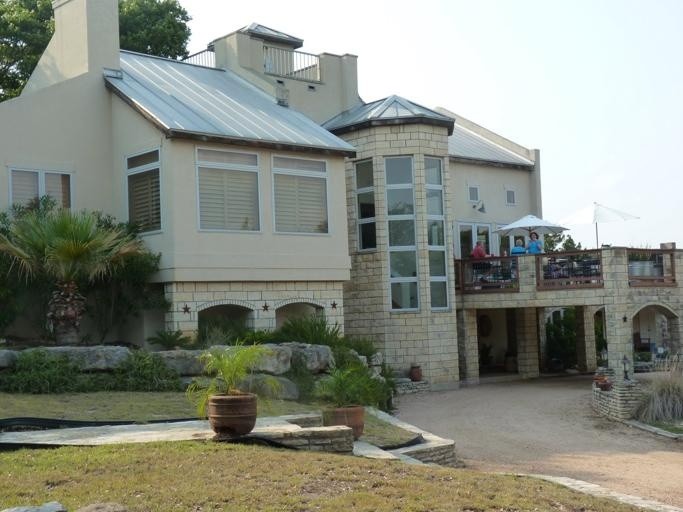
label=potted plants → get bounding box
[316,364,387,442]
[185,337,282,438]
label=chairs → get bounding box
[468,254,492,285]
[583,253,601,284]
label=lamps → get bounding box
[473,199,486,213]
[620,354,631,382]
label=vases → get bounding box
[411,366,422,382]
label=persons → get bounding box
[510,239,526,268]
[525,232,544,254]
[557,254,578,278]
[548,257,561,279]
[472,241,497,284]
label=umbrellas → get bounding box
[492,214,572,241]
